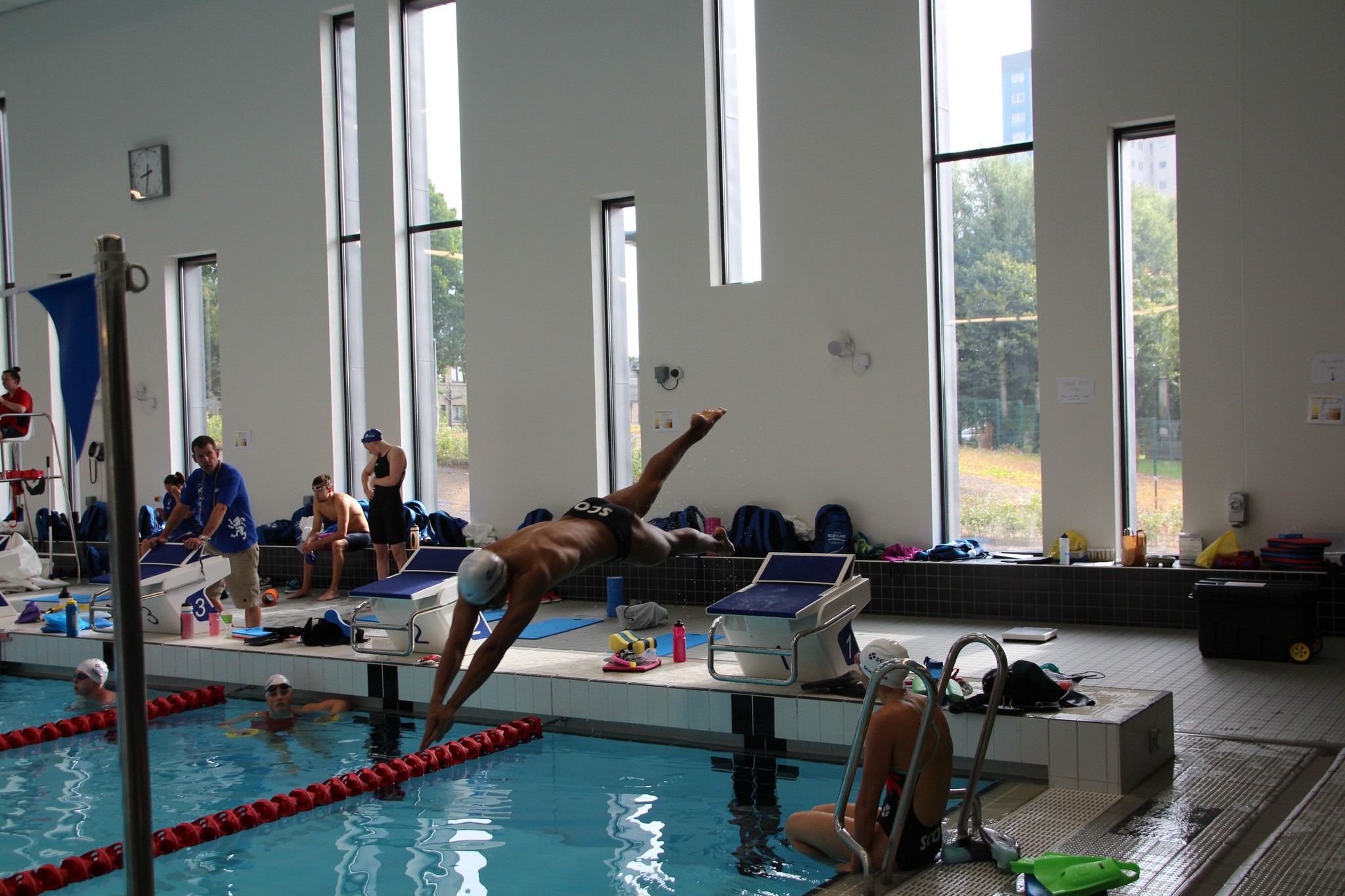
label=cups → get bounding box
[221,610,233,628]
[465,537,474,547]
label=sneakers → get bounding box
[50,568,68,580]
[258,577,273,590]
[284,579,303,593]
[540,591,562,603]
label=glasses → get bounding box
[312,485,327,490]
[265,683,293,694]
[73,672,89,680]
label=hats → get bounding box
[361,429,383,442]
[457,550,508,606]
[77,658,109,685]
[858,644,909,688]
[264,674,292,700]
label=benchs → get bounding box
[22,539,1340,632]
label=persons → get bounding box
[216,673,355,783]
[140,471,203,563]
[0,366,34,445]
[147,434,264,629]
[284,473,372,602]
[359,426,411,609]
[417,405,738,756]
[783,636,955,880]
[64,655,125,741]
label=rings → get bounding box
[191,543,194,546]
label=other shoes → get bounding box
[941,824,1018,870]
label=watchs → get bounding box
[198,533,208,544]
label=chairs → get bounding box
[0,417,33,472]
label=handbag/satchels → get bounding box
[1046,531,1087,560]
[1122,527,1147,566]
[1194,528,1241,569]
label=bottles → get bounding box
[922,655,944,682]
[65,597,79,637]
[1059,532,1070,565]
[59,587,71,607]
[208,606,221,637]
[180,600,195,639]
[305,551,316,564]
[410,523,420,550]
[672,618,687,662]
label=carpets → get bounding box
[353,608,510,623]
[606,631,725,657]
[23,593,113,602]
[513,618,602,639]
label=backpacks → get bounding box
[516,508,553,532]
[78,501,109,541]
[35,508,71,541]
[82,542,109,578]
[915,540,989,561]
[254,500,428,548]
[427,511,468,547]
[646,505,801,557]
[3,504,23,522]
[809,504,853,554]
[137,505,161,540]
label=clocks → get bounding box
[127,145,172,204]
